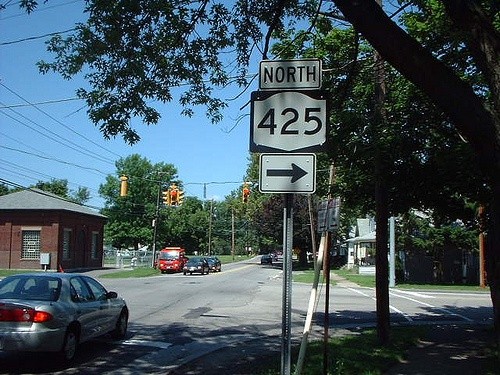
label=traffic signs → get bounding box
[259,58,322,92]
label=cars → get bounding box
[0,274,128,364]
[261,256,272,264]
[183,257,209,274]
[206,256,221,272]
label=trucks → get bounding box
[158,247,189,273]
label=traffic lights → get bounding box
[163,189,171,205]
[169,190,177,207]
[243,189,249,202]
[177,191,184,206]
